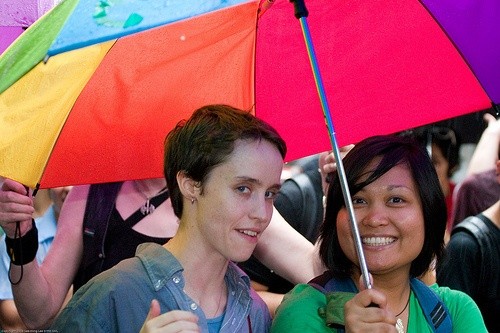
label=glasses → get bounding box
[422,127,456,145]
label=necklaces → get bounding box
[383,282,421,333]
[133,185,169,215]
[212,290,224,320]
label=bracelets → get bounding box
[6,220,40,266]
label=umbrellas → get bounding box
[0,0,500,289]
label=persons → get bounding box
[270,134,487,333]
[46,105,287,333]
[413,111,500,333]
[0,143,356,333]
